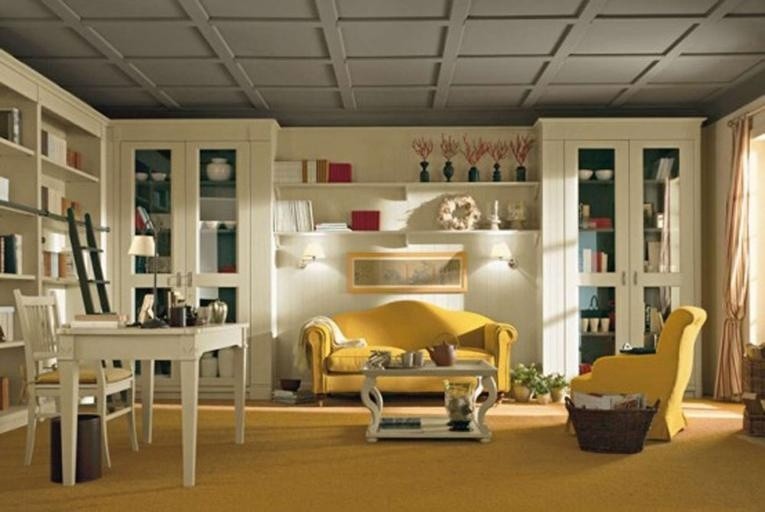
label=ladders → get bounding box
[67,207,129,414]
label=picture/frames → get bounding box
[345,251,469,293]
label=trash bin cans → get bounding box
[50,414,102,484]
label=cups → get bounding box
[395,350,423,369]
[581,318,611,333]
[171,307,187,328]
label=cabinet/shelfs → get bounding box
[112,118,279,401]
[533,115,707,400]
[0,48,112,436]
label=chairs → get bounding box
[566,306,707,442]
[12,288,140,468]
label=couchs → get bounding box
[299,300,518,406]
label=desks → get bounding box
[55,320,250,487]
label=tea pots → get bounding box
[207,297,228,324]
[424,331,460,366]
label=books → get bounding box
[582,249,608,273]
[1,234,22,274]
[651,157,675,180]
[380,417,421,429]
[276,200,353,232]
[71,314,125,328]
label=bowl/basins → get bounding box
[150,173,167,182]
[595,169,613,181]
[135,172,148,182]
[205,220,221,230]
[222,221,236,230]
[579,169,594,180]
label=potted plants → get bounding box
[275,181,542,248]
[508,361,565,405]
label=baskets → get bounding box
[742,352,765,398]
[564,397,660,454]
[743,408,765,436]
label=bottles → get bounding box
[200,352,218,377]
[206,159,233,181]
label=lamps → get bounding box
[127,218,166,329]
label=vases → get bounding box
[419,160,526,182]
